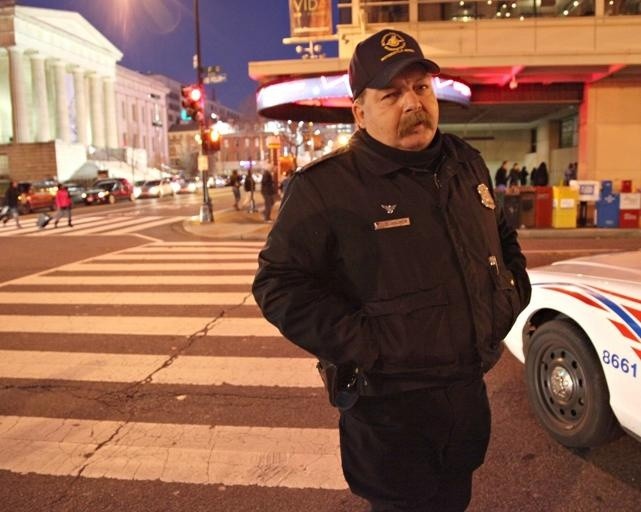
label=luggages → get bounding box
[36,212,52,228]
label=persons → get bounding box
[280,169,295,197]
[496,156,549,193]
[226,170,243,210]
[53,183,74,228]
[564,164,574,185]
[4,180,24,228]
[260,169,276,222]
[253,30,533,512]
[244,169,259,212]
[571,163,579,184]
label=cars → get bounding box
[501,249,641,451]
[1,173,263,215]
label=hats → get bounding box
[348,29,440,101]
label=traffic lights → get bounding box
[181,84,196,116]
[202,129,220,152]
[191,88,203,121]
